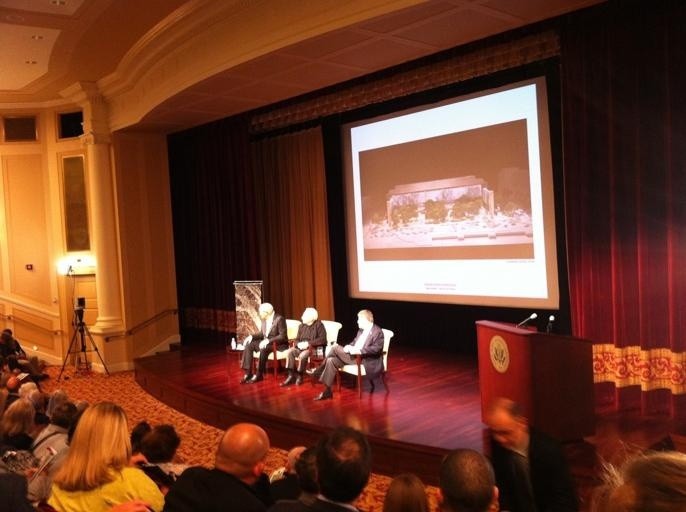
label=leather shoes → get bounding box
[239,368,332,401]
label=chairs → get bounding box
[253,318,302,384]
[294,320,342,386]
[336,328,393,399]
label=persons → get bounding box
[587,438,686,512]
[434,447,499,512]
[238,300,288,385]
[279,306,328,388]
[2,327,320,512]
[273,425,373,511]
[382,472,429,512]
[485,397,580,512]
[304,310,385,402]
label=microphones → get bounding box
[538,316,558,331]
[510,309,538,325]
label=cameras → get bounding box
[72,293,89,314]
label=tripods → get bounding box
[55,321,112,381]
